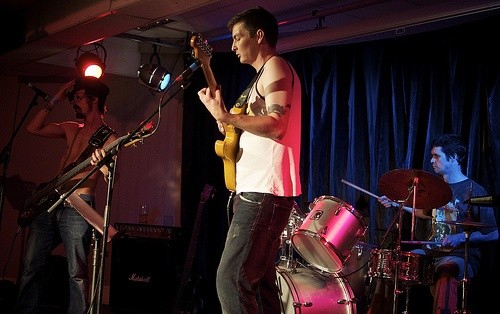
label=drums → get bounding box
[275,265,357,314]
[369,249,396,280]
[295,198,369,275]
[398,251,425,282]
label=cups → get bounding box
[138,199,151,225]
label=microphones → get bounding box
[170,59,201,84]
[26,81,52,103]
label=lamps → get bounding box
[135,45,172,95]
[73,40,107,82]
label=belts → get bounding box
[57,201,95,209]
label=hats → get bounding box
[68,76,110,100]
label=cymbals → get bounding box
[379,168,453,209]
[436,220,496,228]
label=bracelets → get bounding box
[52,95,58,104]
[43,102,55,114]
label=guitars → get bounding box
[17,118,155,227]
[170,182,217,314]
[190,30,248,192]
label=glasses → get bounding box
[70,95,87,104]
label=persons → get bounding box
[198,5,303,313]
[378,132,498,314]
[16,76,121,314]
